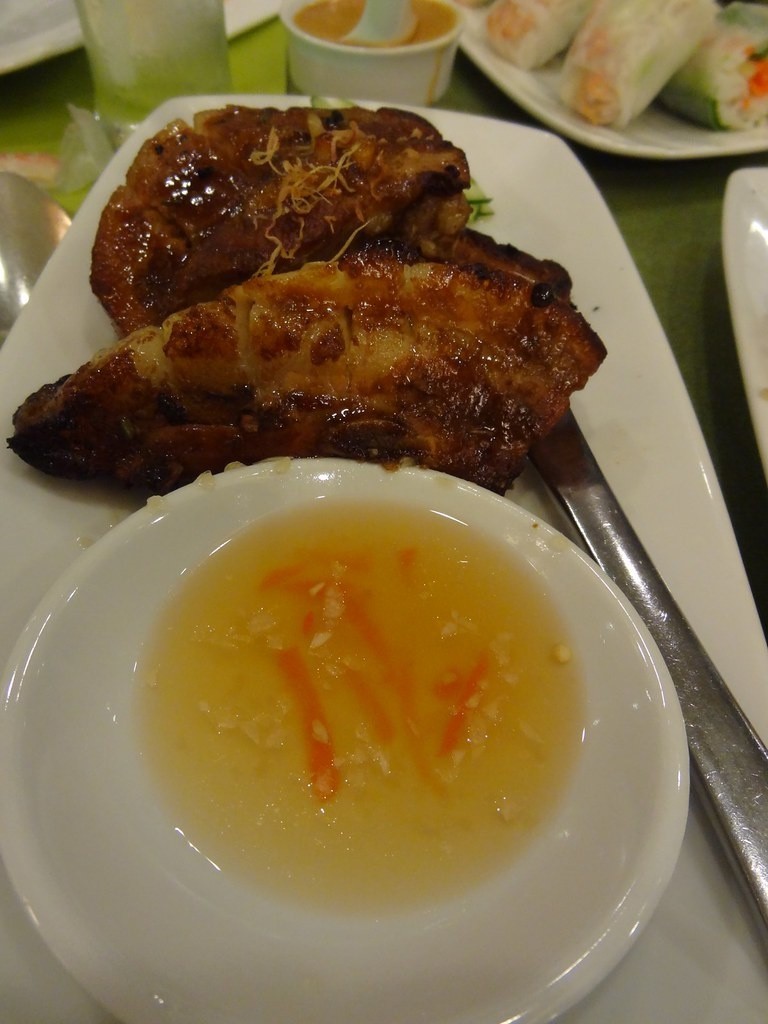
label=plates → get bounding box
[0,93,768,1024]
[455,1,768,158]
[0,0,302,73]
[721,168,768,477]
[1,461,685,1024]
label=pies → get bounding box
[457,0,768,130]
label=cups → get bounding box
[268,0,461,105]
[75,0,233,141]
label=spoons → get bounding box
[0,172,75,335]
